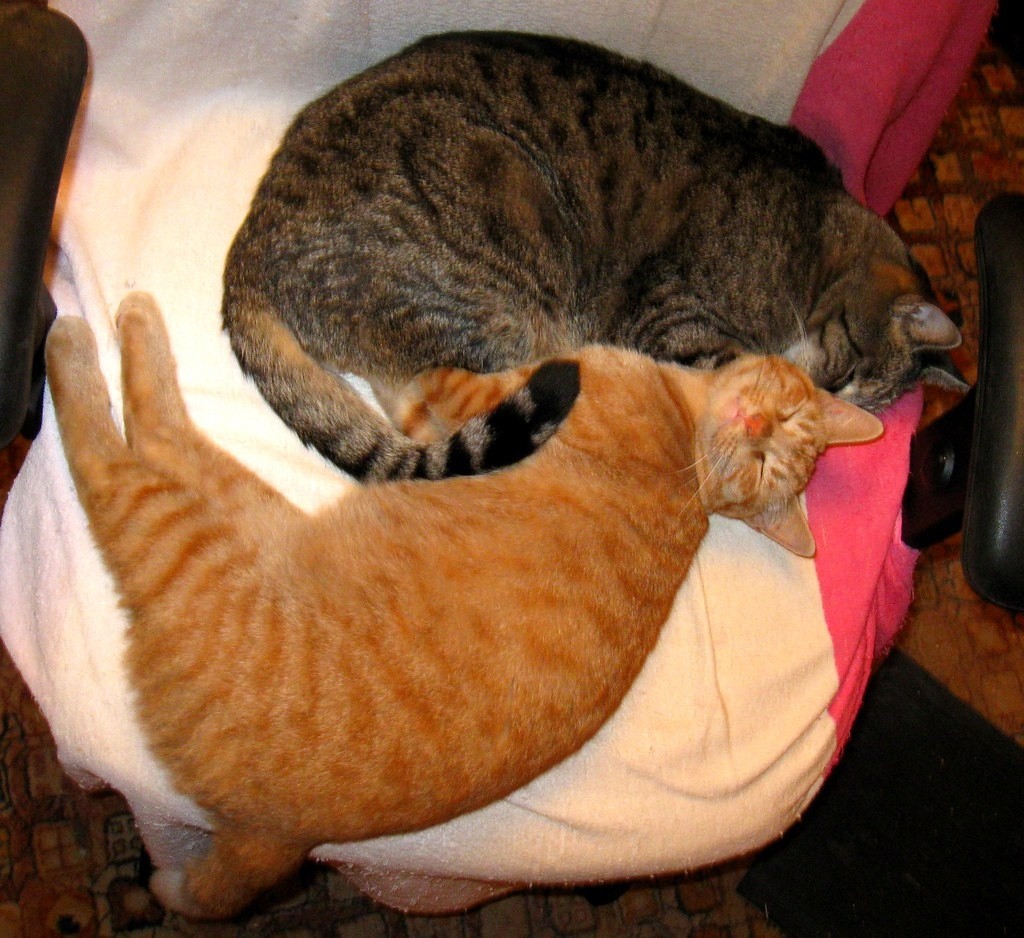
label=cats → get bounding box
[43,30,969,908]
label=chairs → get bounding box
[0,0,1024,938]
